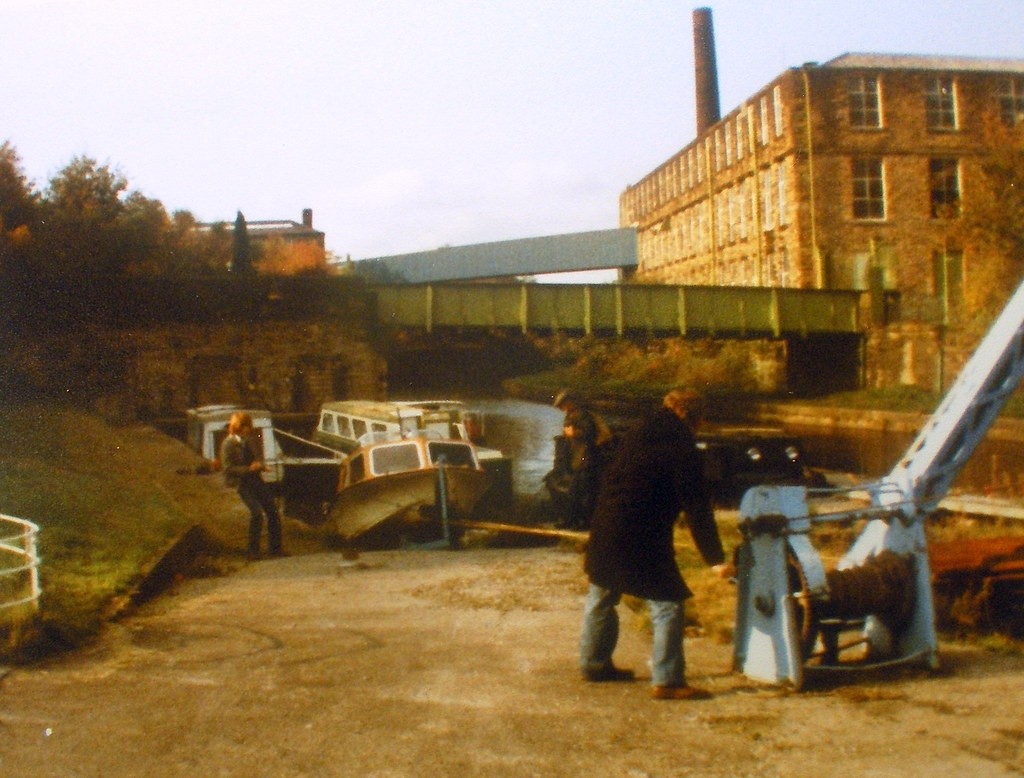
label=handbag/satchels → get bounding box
[544,467,579,502]
[586,411,613,446]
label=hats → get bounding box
[553,389,572,407]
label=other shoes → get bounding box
[650,685,709,699]
[268,549,290,556]
[248,551,261,560]
[583,668,635,681]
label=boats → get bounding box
[187,401,511,547]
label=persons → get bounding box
[221,411,292,559]
[578,386,735,700]
[542,387,613,532]
